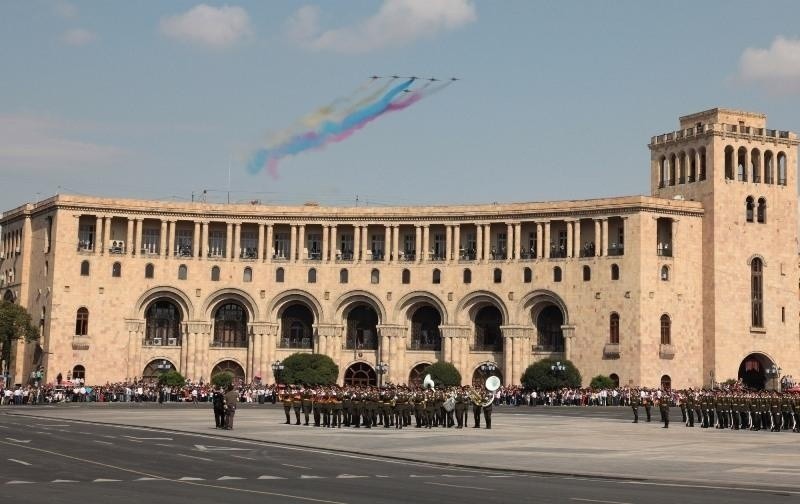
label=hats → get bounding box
[632,386,800,396]
[279,379,482,394]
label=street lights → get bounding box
[481,361,495,380]
[766,364,782,391]
[550,360,567,388]
[376,360,390,389]
[157,360,171,373]
[272,359,285,385]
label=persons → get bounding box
[0,367,800,405]
[520,246,536,258]
[632,389,800,433]
[213,385,239,430]
[471,248,477,260]
[281,384,493,430]
[490,246,508,260]
[584,241,595,257]
[414,333,440,350]
[550,242,566,258]
[431,246,435,261]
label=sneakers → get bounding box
[630,418,800,434]
[282,418,493,430]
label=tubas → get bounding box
[468,386,482,407]
